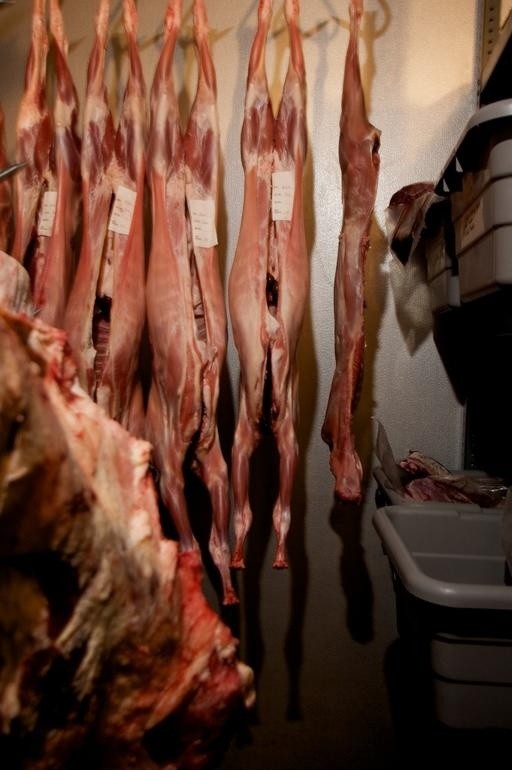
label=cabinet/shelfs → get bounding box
[460,4,512,472]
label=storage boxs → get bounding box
[371,466,510,513]
[373,505,512,768]
[411,101,511,411]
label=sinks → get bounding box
[375,503,510,611]
